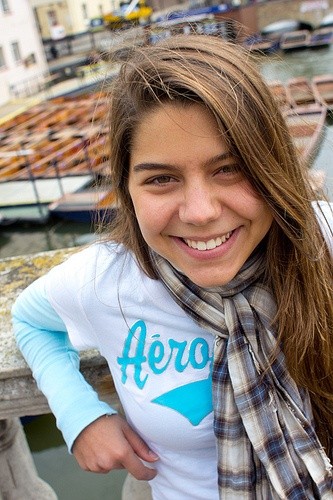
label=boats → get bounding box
[1,72,333,225]
[244,12,333,55]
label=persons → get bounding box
[12,32,333,500]
